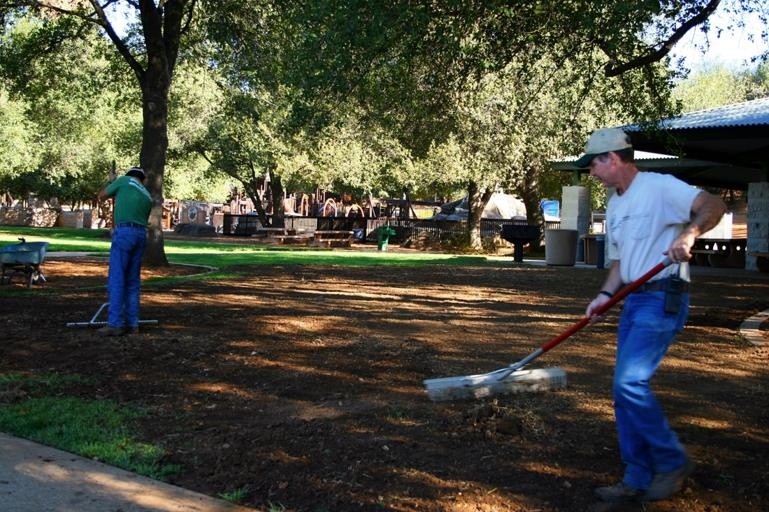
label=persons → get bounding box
[97,166,152,337]
[573,125,727,511]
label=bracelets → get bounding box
[598,291,613,298]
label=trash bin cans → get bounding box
[378,225,390,251]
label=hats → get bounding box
[125,166,143,178]
[574,128,632,168]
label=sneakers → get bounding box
[646,462,691,503]
[103,325,125,336]
[127,328,139,334]
[594,484,642,502]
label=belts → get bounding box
[115,221,142,229]
[630,280,689,295]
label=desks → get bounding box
[313,231,354,239]
[258,228,289,237]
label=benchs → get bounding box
[251,234,351,247]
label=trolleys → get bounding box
[1,241,46,286]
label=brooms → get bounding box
[67,161,160,332]
[425,253,675,401]
